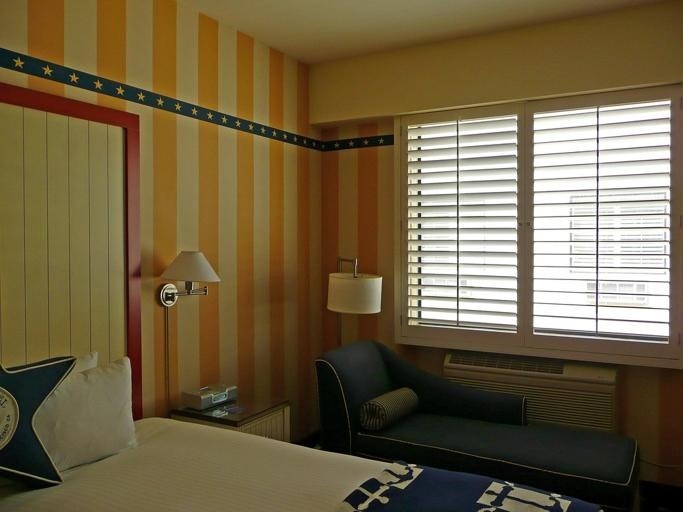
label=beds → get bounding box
[0,351,604,512]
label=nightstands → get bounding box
[171,397,292,444]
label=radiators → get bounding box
[442,350,618,431]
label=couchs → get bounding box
[313,339,639,507]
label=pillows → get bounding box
[32,355,137,473]
[69,352,98,373]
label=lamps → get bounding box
[326,255,382,346]
[157,251,220,309]
